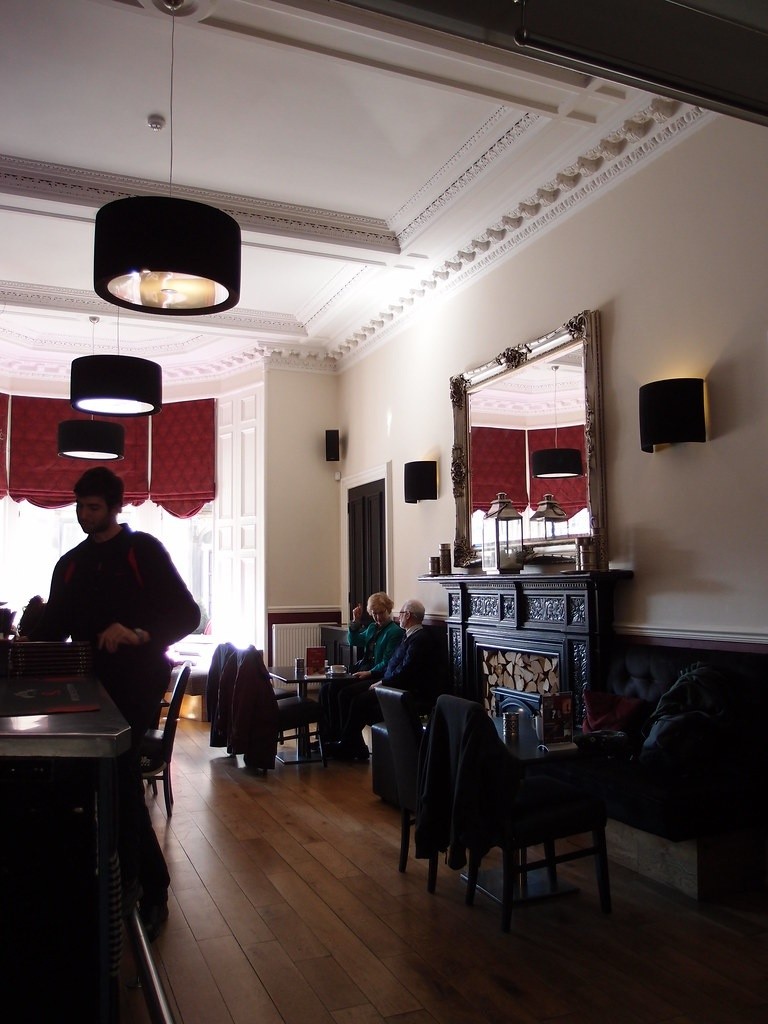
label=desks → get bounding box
[458,717,625,905]
[0,671,132,1024]
[266,666,364,765]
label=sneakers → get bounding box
[141,754,166,777]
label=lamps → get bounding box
[325,426,340,460]
[532,359,582,480]
[69,302,163,415]
[639,377,707,455]
[59,313,127,460]
[94,0,240,317]
[403,460,438,503]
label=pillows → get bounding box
[580,688,647,734]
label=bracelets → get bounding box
[134,628,144,645]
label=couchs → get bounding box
[580,642,768,900]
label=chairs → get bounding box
[414,693,616,928]
[374,683,490,895]
[139,667,190,817]
[205,643,327,769]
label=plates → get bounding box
[559,569,586,574]
[325,666,330,668]
[328,671,347,676]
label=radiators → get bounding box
[272,621,338,690]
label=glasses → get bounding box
[399,611,412,615]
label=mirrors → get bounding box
[451,307,599,570]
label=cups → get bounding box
[325,660,328,666]
[575,537,597,571]
[503,712,520,732]
[532,715,543,742]
[429,556,439,576]
[330,664,347,674]
[439,544,451,575]
[295,658,304,669]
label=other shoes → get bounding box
[324,739,353,759]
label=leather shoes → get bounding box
[136,895,168,940]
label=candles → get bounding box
[500,547,506,563]
[491,551,496,566]
[510,548,517,561]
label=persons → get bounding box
[17,467,201,942]
[310,591,404,748]
[324,600,433,759]
